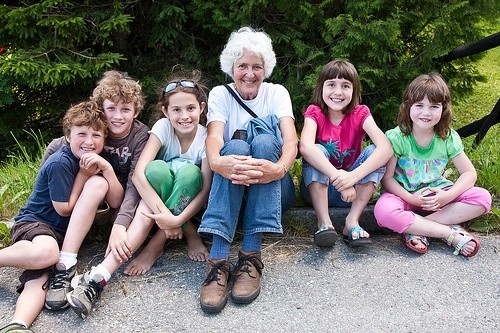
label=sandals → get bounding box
[403,233,429,253]
[442,224,481,256]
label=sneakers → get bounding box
[42,263,78,311]
[66,269,108,320]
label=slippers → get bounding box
[314,225,338,247]
[343,226,373,247]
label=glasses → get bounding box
[162,79,199,98]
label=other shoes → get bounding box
[0,323,35,333]
[232,249,264,304]
[199,257,234,314]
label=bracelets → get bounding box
[277,161,287,175]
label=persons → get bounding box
[299,60,394,246]
[200,26,298,314]
[0,101,124,333]
[123,65,214,276]
[40,71,151,318]
[374,74,492,256]
